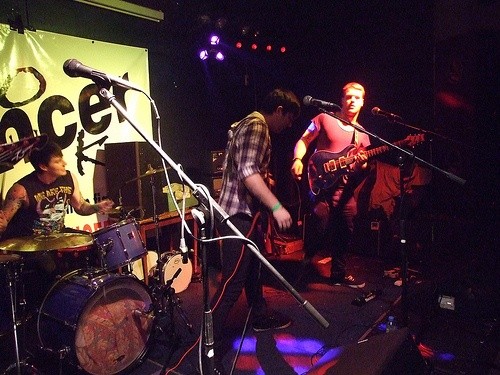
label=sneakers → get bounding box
[202,354,228,375]
[333,274,366,289]
[296,275,311,292]
[251,309,292,332]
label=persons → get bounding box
[0,140,114,238]
[361,140,430,273]
[292,82,370,289]
[210,89,300,375]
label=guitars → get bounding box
[307,133,425,196]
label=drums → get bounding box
[92,218,148,272]
[0,254,24,285]
[36,264,158,375]
[155,251,193,294]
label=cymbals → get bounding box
[125,167,172,184]
[0,232,94,252]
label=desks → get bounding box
[107,206,199,287]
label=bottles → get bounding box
[387,316,398,331]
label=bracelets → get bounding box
[292,158,302,165]
[271,202,281,211]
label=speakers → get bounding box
[304,327,433,375]
[104,141,169,220]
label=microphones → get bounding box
[132,310,154,319]
[371,107,403,120]
[302,95,341,110]
[178,237,189,264]
[63,58,144,92]
